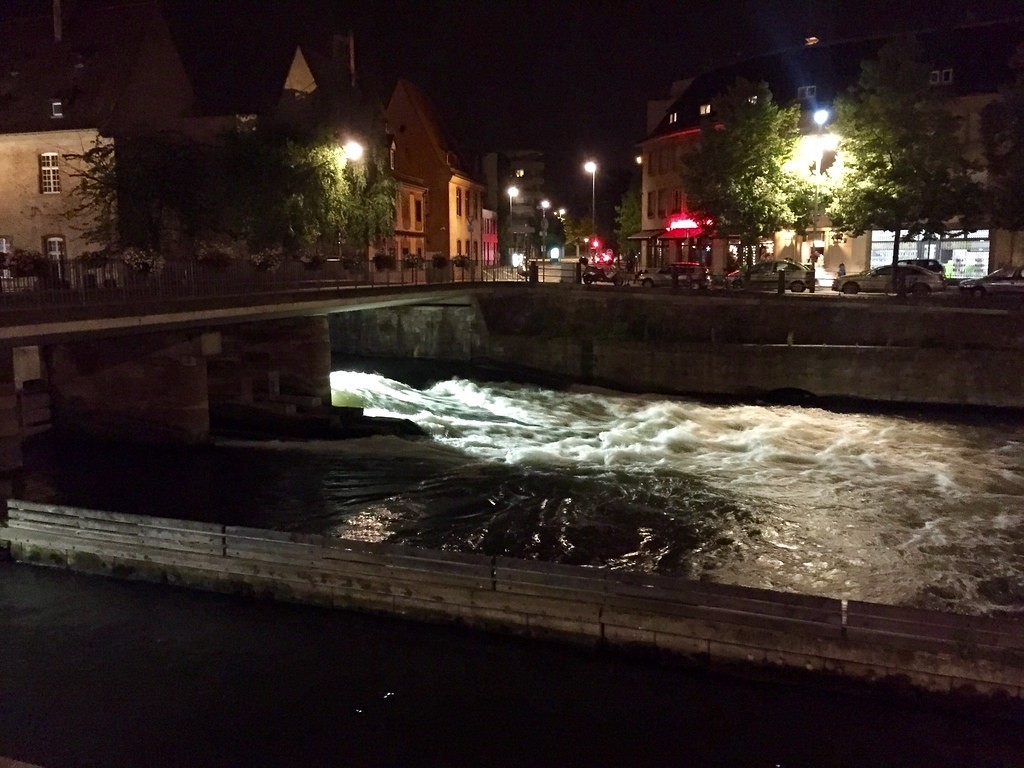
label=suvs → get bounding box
[897,259,944,278]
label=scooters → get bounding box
[579,249,624,287]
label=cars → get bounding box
[831,264,943,296]
[721,257,815,293]
[637,262,712,292]
[959,264,1024,297]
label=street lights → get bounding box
[584,160,597,265]
[541,200,550,283]
[811,108,829,269]
[338,141,362,261]
[507,186,519,280]
[558,209,566,262]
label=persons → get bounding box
[838,263,846,276]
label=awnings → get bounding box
[658,228,705,239]
[627,230,666,240]
